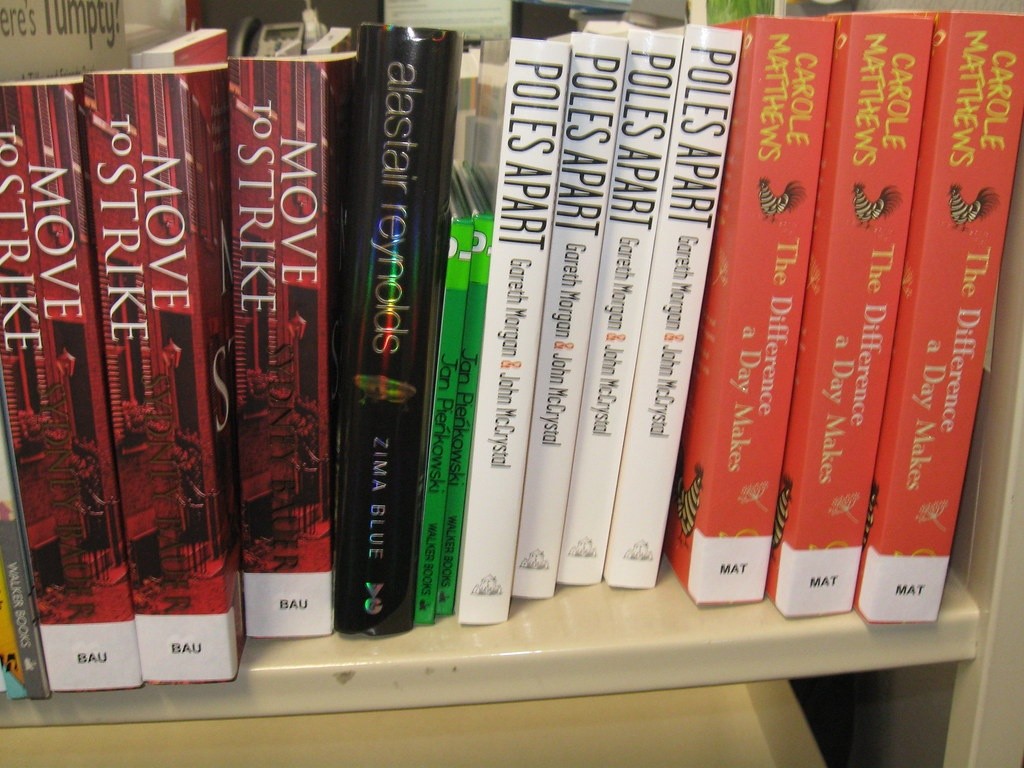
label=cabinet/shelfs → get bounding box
[0,159,1024,768]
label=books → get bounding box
[0,6,1024,699]
